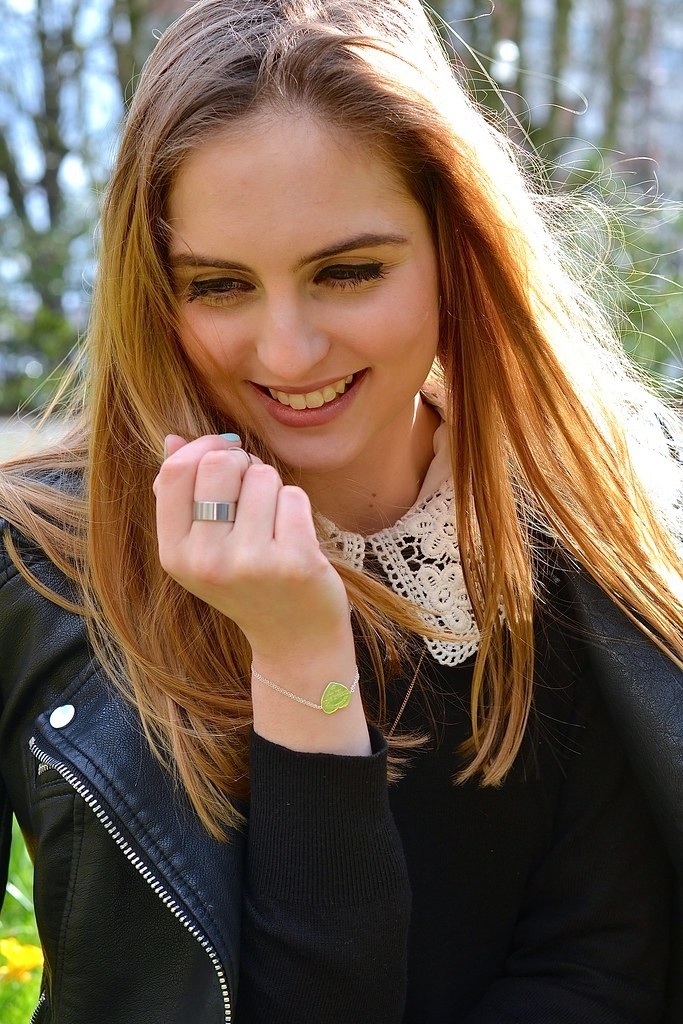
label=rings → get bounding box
[228,447,253,469]
[191,503,238,522]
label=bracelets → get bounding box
[252,663,360,714]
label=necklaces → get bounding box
[386,646,427,739]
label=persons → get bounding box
[0,0,683,1024]
